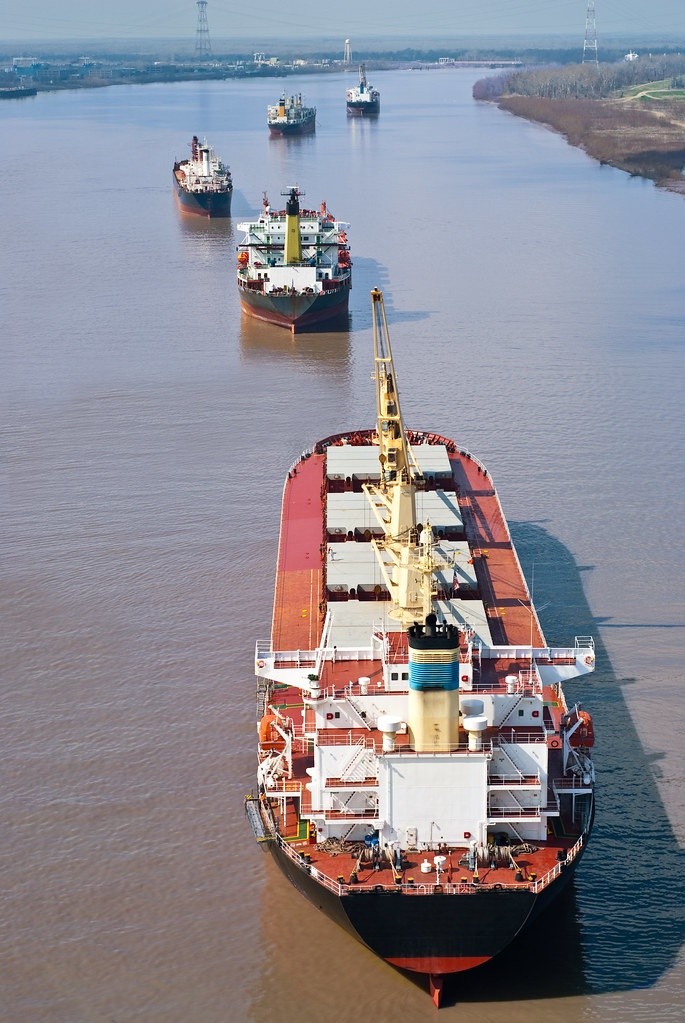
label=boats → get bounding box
[236,182,353,333]
[267,90,316,136]
[346,64,380,114]
[173,136,233,219]
[244,285,595,1008]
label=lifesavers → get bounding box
[551,740,559,747]
